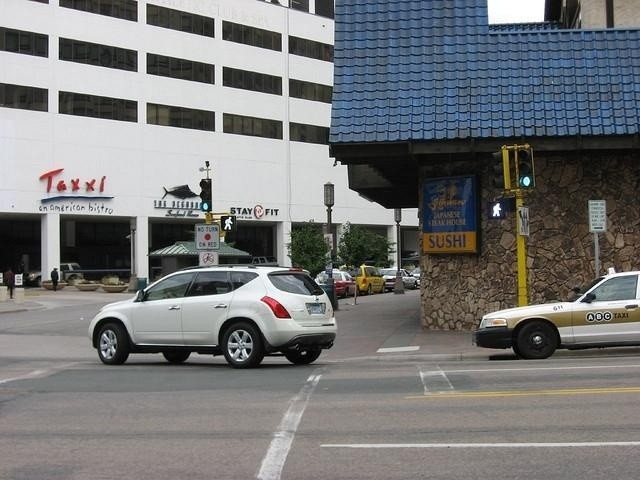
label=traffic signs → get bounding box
[589,199,606,232]
[196,223,220,250]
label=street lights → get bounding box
[392,209,405,295]
[323,180,336,310]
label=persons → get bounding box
[4,269,14,300]
[51,267,58,292]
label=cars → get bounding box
[411,269,420,287]
[314,269,356,297]
[381,269,418,290]
[475,271,640,359]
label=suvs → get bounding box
[341,265,385,295]
[24,263,84,287]
[88,263,338,368]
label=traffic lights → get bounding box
[199,178,212,212]
[489,202,505,221]
[220,216,236,232]
[517,146,536,192]
[493,147,505,179]
